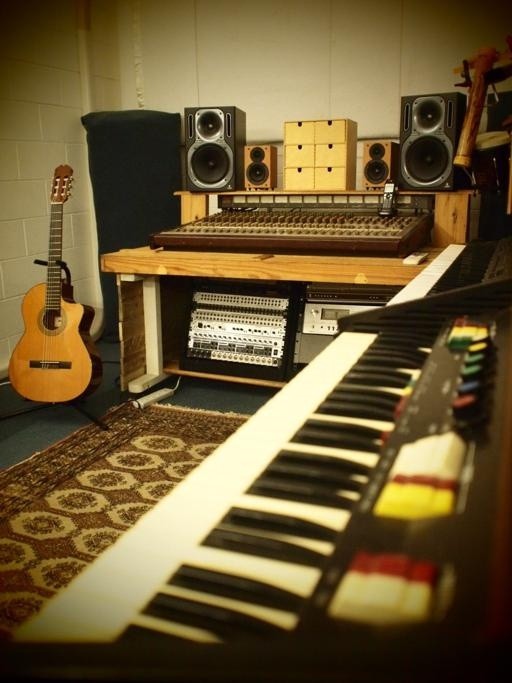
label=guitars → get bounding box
[9,165,103,404]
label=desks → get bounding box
[99,186,483,398]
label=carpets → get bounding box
[0,392,254,653]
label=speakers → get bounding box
[244,144,277,189]
[184,106,246,192]
[399,92,467,191]
[362,141,399,188]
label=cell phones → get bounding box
[380,179,398,216]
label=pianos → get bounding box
[14,277,509,643]
[384,236,511,306]
